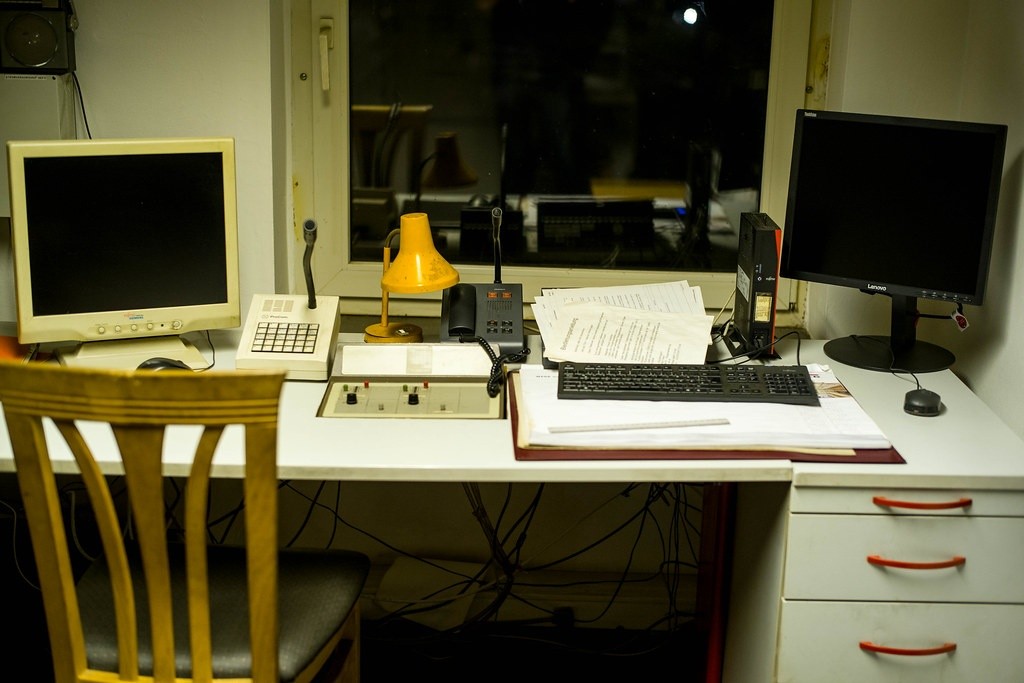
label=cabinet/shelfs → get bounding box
[720,482,1024,683]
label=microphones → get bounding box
[491,206,502,284]
[497,124,511,211]
[301,218,317,309]
[370,102,403,196]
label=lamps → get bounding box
[405,132,479,212]
[366,212,461,344]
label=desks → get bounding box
[1,329,1024,683]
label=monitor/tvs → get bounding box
[779,109,1009,374]
[7,137,241,372]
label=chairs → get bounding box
[1,354,371,683]
[350,103,435,195]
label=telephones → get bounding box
[439,283,525,362]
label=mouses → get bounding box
[136,357,193,372]
[904,388,941,416]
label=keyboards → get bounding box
[558,361,819,405]
[538,215,654,252]
